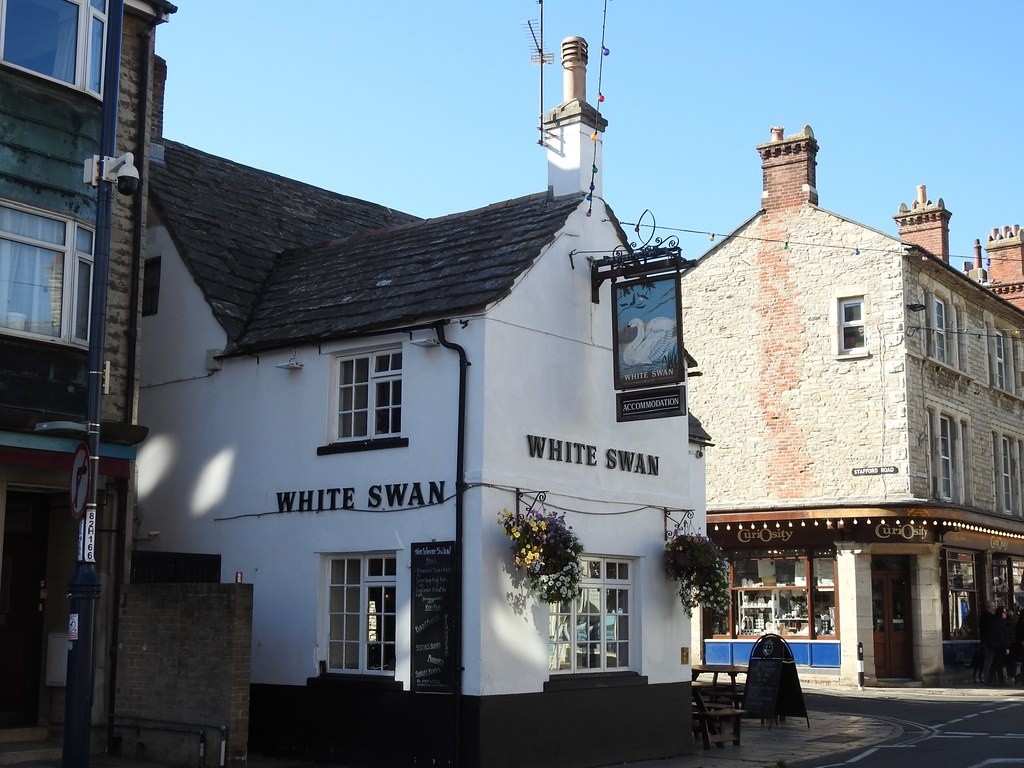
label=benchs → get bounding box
[692,683,762,738]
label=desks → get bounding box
[686,664,752,708]
[689,681,733,750]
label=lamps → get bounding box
[907,303,927,311]
[275,349,304,370]
[134,529,161,543]
[410,338,441,347]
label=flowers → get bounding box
[498,509,587,604]
[665,534,734,620]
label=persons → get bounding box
[979,601,1024,689]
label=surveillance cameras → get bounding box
[115,162,140,196]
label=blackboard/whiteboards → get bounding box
[741,633,810,718]
[409,540,456,700]
